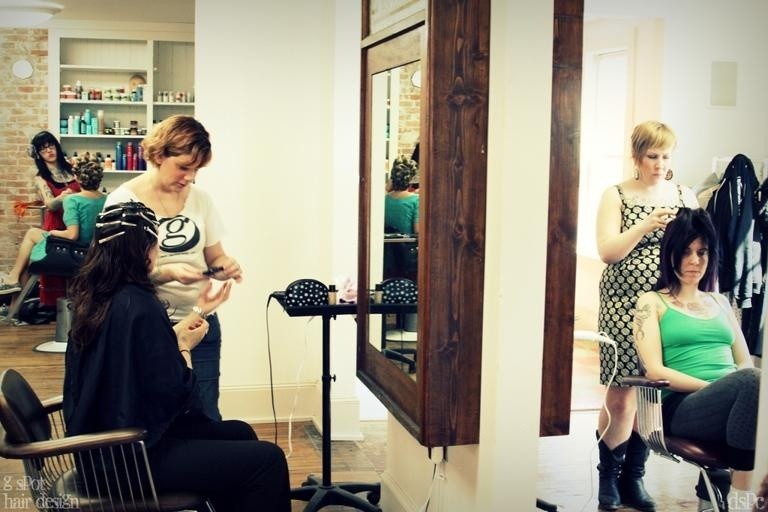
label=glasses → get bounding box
[38,143,56,153]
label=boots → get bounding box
[593,428,628,512]
[620,430,659,512]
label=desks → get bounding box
[382,294,422,373]
[268,296,387,512]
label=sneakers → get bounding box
[0,282,22,295]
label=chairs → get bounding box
[619,376,726,512]
[1,368,214,511]
[1,235,92,355]
[381,225,416,347]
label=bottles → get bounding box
[63,141,146,171]
[374,284,384,305]
[326,284,338,306]
[66,107,164,136]
[59,78,195,104]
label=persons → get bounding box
[630,207,761,511]
[62,198,290,509]
[407,140,418,192]
[106,113,241,427]
[596,119,700,512]
[384,153,418,272]
[30,131,74,308]
[2,157,109,318]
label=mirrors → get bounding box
[350,1,497,447]
[534,1,766,510]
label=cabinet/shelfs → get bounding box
[44,20,194,175]
